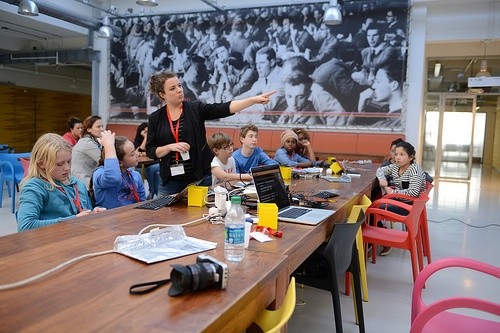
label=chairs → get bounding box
[0,152,31,213]
[246,181,500,333]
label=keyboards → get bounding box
[133,195,174,210]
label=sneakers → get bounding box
[367,246,372,258]
[380,246,392,255]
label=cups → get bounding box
[245,218,253,248]
[180,151,190,161]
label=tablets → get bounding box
[165,179,204,207]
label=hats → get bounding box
[280,129,298,146]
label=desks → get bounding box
[0,163,384,333]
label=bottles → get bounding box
[224,196,245,261]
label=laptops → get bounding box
[249,163,336,226]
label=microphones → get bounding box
[167,253,229,297]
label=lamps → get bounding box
[323,0,343,25]
[97,15,113,38]
[475,42,492,77]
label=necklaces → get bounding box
[166,103,185,176]
[122,169,140,203]
[42,177,82,213]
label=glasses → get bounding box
[298,137,307,141]
[217,143,234,150]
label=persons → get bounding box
[231,124,279,174]
[368,142,426,256]
[134,122,163,200]
[17,133,107,233]
[208,132,253,186]
[71,115,104,190]
[62,116,83,146]
[88,130,146,210]
[111,0,410,126]
[145,72,278,199]
[292,128,316,161]
[381,138,434,184]
[274,129,317,168]
